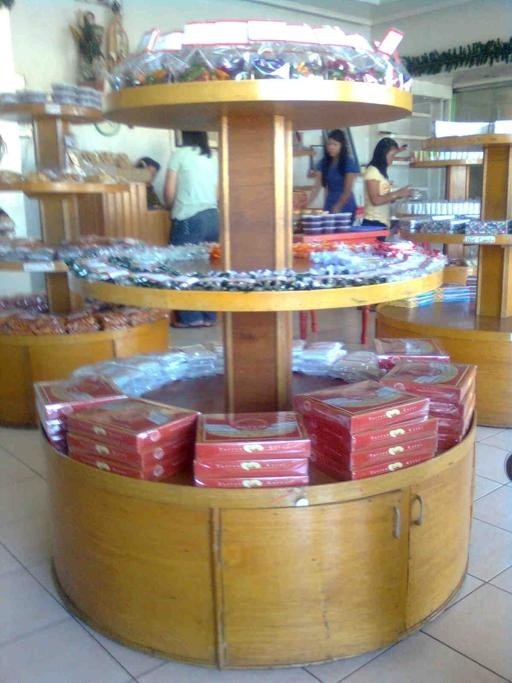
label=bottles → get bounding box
[395,198,480,218]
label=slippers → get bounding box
[170,320,204,327]
[203,320,213,326]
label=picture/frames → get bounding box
[309,145,328,171]
[175,127,220,149]
[321,125,359,172]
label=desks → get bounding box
[294,230,389,344]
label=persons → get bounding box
[360,137,414,242]
[162,130,221,329]
[307,128,358,226]
[135,157,163,209]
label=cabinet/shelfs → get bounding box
[0,99,170,427]
[41,76,477,670]
[365,79,451,199]
[375,133,512,431]
[395,157,483,260]
